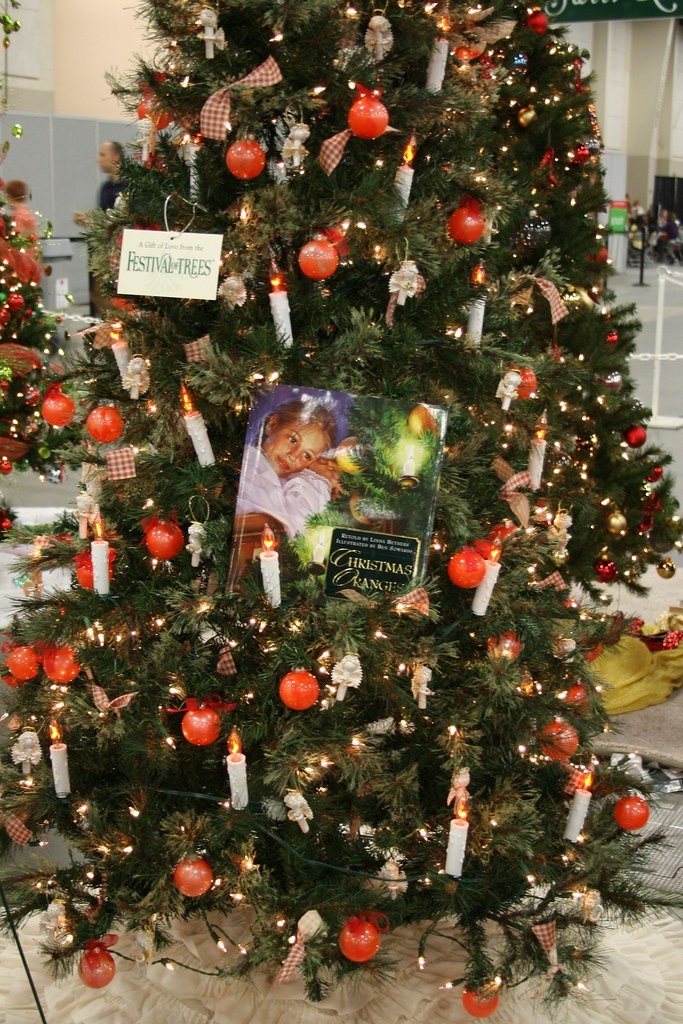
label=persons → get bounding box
[235,399,343,542]
[626,194,683,266]
[98,141,128,211]
